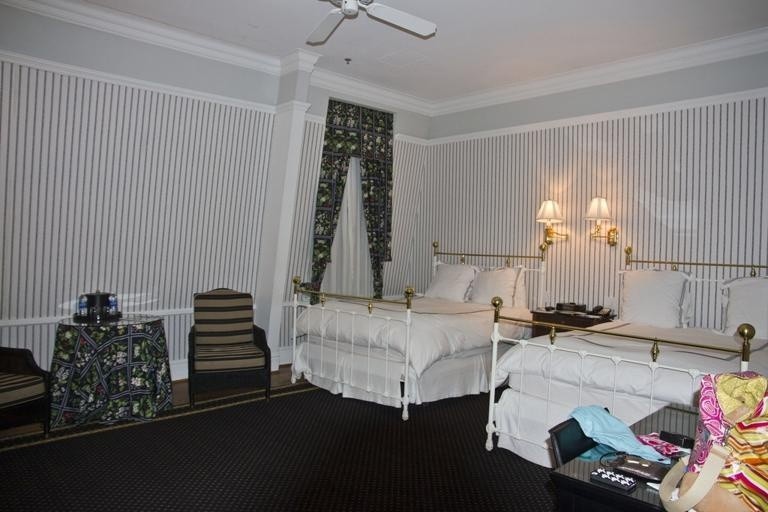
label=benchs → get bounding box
[187,287,271,407]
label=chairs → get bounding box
[0,347,52,439]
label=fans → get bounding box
[302,1,437,43]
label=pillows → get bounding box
[424,262,480,303]
[472,266,525,308]
[622,270,692,330]
[710,277,768,340]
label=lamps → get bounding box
[537,196,618,246]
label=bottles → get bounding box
[78,292,88,316]
[107,293,118,315]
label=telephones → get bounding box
[592,306,611,316]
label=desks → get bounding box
[550,402,699,511]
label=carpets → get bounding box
[0,379,572,512]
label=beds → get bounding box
[486,246,768,468]
[292,242,547,421]
